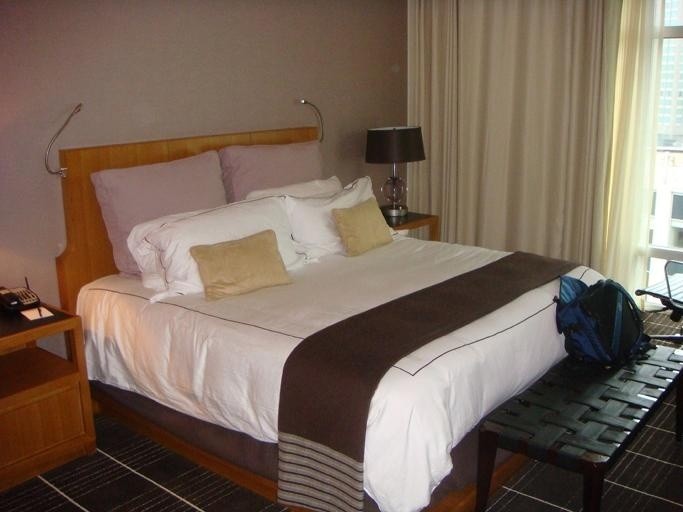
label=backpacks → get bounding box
[557,280,647,373]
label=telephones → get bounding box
[0,277,39,313]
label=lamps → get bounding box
[363,127,426,216]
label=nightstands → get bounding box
[0,301,98,493]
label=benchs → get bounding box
[475,340,683,512]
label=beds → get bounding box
[54,126,606,512]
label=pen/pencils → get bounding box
[37,306,41,316]
